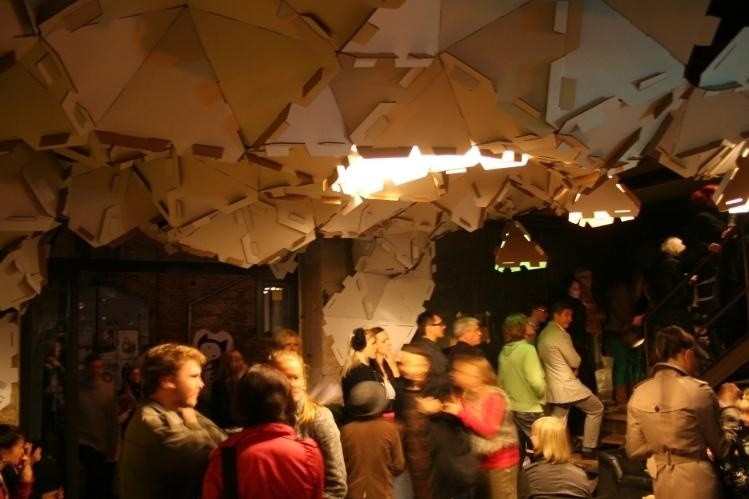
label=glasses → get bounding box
[429,322,446,327]
[537,308,546,313]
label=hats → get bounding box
[350,381,394,419]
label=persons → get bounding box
[640,236,698,324]
[0,327,407,499]
[692,185,746,350]
[397,266,749,499]
